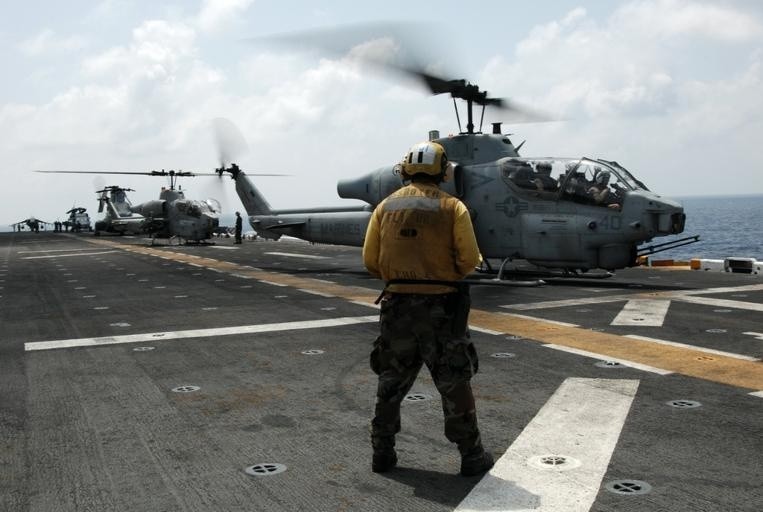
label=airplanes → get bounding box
[93,185,146,238]
[63,207,93,233]
[9,217,52,235]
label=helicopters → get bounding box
[225,65,709,288]
[33,170,297,248]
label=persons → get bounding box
[512,161,622,210]
[363,143,495,477]
[234,212,242,244]
[54,221,81,233]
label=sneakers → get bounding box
[371,448,396,472]
[460,452,494,469]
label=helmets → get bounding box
[392,141,450,186]
